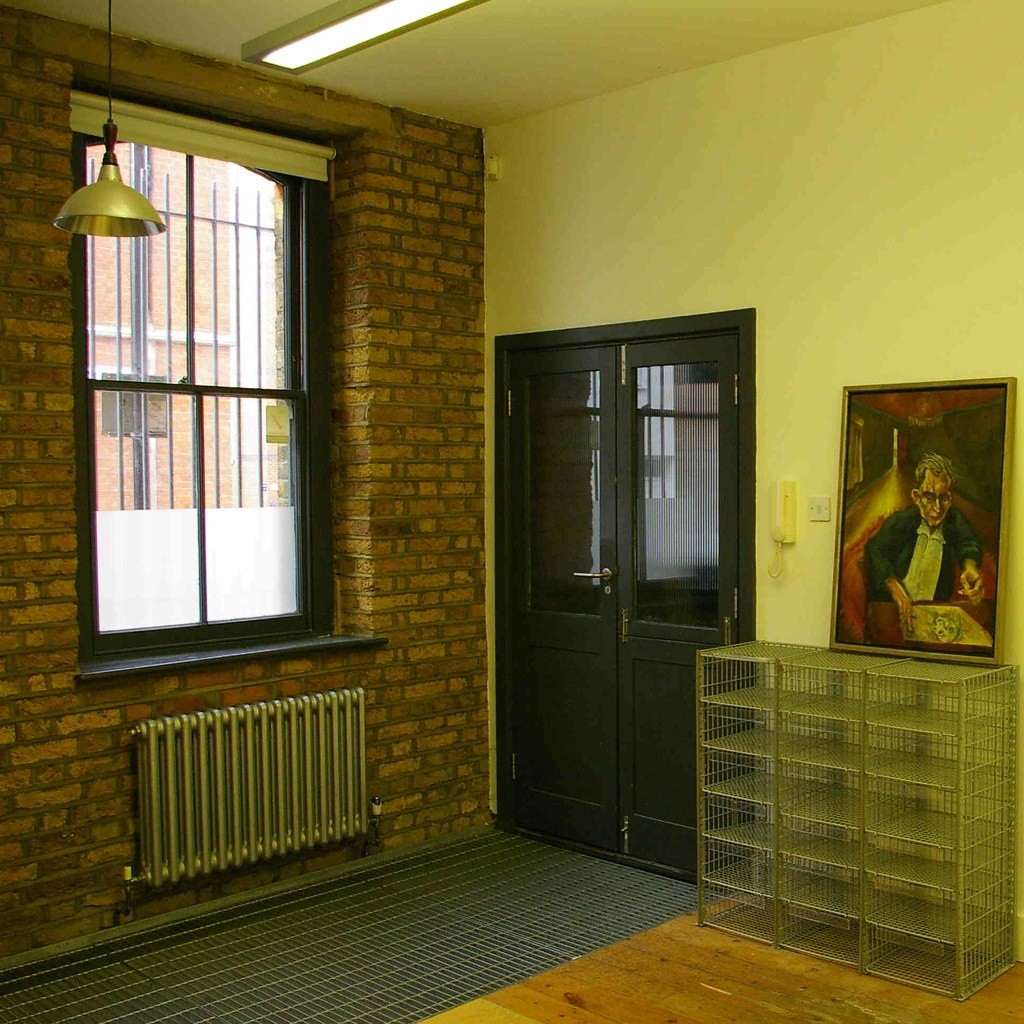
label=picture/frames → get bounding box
[829,376,1016,665]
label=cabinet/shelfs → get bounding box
[694,639,1018,1002]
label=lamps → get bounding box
[241,0,491,75]
[53,0,167,237]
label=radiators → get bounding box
[118,687,383,914]
